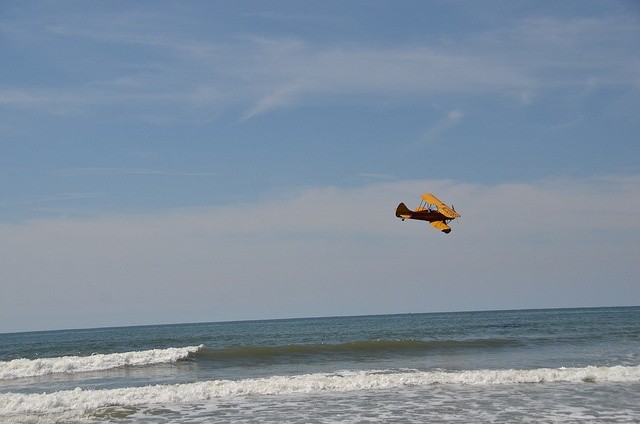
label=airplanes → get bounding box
[395,193,461,234]
